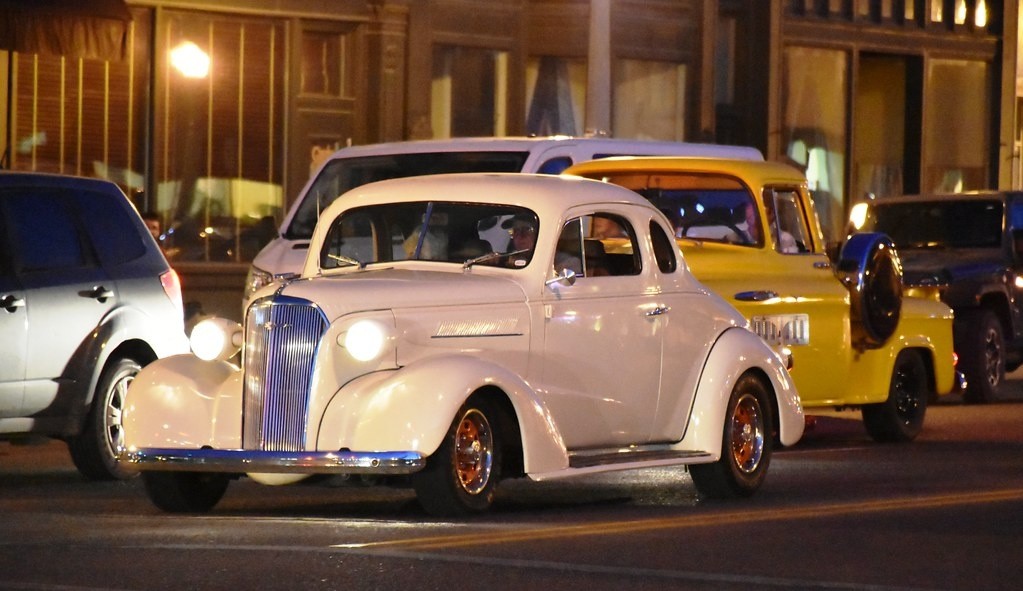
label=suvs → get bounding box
[0,171,191,481]
[839,189,1023,404]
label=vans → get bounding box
[243,137,768,322]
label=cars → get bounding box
[116,171,816,519]
[154,213,263,260]
[560,157,960,442]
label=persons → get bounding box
[720,192,799,253]
[498,210,586,278]
[142,210,162,240]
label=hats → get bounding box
[501,213,538,229]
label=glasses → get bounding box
[507,226,534,239]
